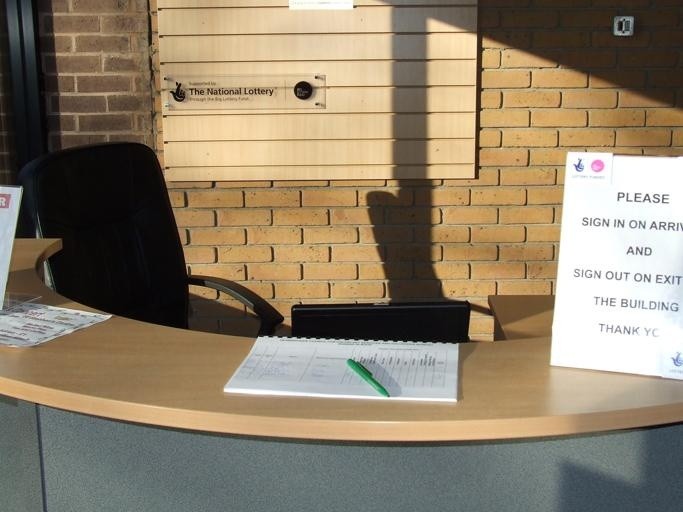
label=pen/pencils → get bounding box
[347,359,390,398]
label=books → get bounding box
[221,334,461,405]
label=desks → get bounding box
[0,241,682,507]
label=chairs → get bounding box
[18,142,285,336]
[290,301,471,340]
